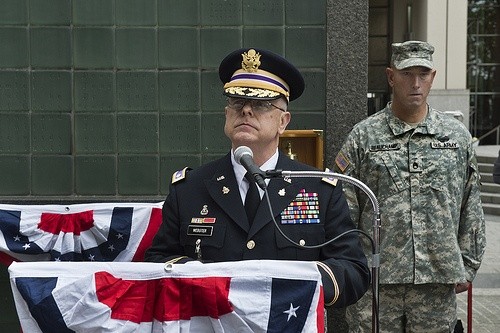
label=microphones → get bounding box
[234,145,266,190]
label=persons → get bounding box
[332,40,486,333]
[144,46,372,306]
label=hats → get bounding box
[390,41,434,70]
[219,48,304,105]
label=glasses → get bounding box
[228,99,285,112]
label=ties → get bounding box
[245,172,262,226]
